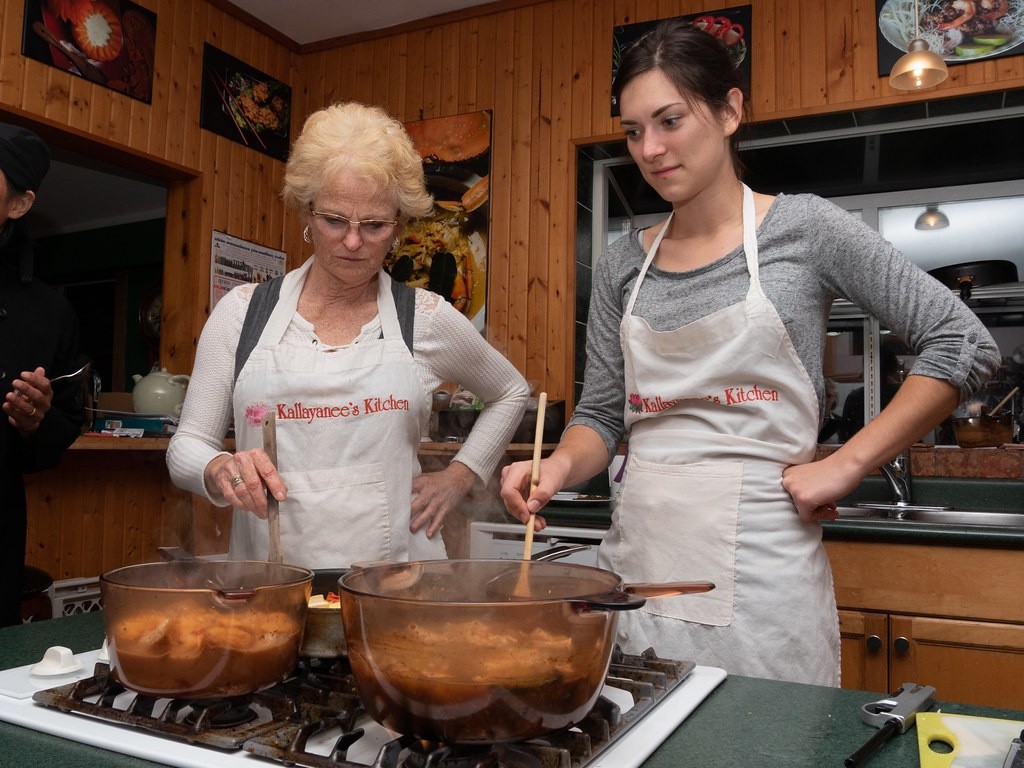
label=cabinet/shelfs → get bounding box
[821,540,1024,713]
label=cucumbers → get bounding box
[955,33,1010,56]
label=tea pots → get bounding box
[131,367,191,418]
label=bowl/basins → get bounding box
[952,411,1013,448]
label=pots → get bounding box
[335,542,717,746]
[99,546,315,707]
[299,567,349,659]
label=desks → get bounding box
[69,436,560,455]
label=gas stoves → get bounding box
[0,635,729,768]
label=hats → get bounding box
[0,124,52,195]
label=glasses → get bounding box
[308,201,397,243]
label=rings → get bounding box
[30,408,38,417]
[231,475,244,488]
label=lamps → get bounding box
[916,205,949,229]
[889,0,948,90]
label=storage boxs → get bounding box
[438,400,565,443]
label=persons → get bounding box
[0,119,94,630]
[497,21,1000,690]
[162,104,532,572]
[818,352,905,447]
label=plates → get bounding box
[879,0,1024,61]
[550,493,617,502]
[422,163,488,335]
[633,22,746,68]
